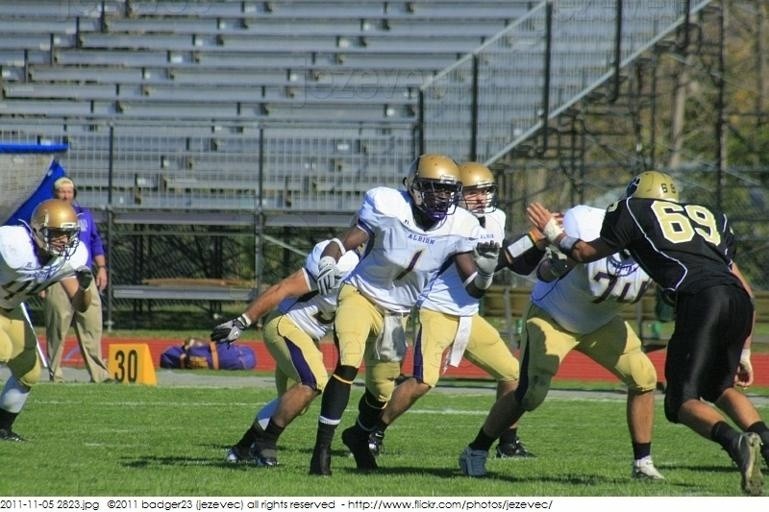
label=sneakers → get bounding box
[89,379,115,383]
[727,429,769,496]
[225,436,280,469]
[309,442,332,476]
[458,444,489,478]
[632,454,666,480]
[495,437,539,458]
[341,422,386,473]
[0,426,28,444]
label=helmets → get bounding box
[625,171,679,204]
[30,199,81,261]
[457,162,498,214]
[403,153,463,222]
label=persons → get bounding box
[309,153,501,477]
[181,336,195,352]
[458,203,663,480]
[1,200,80,440]
[526,171,769,497]
[350,162,564,459]
[38,178,118,383]
[210,237,368,466]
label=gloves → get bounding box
[209,313,252,350]
[74,265,93,292]
[472,240,501,278]
[316,261,343,297]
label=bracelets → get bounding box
[559,235,582,256]
[97,265,107,269]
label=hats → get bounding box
[53,176,77,199]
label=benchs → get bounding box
[0,0,710,302]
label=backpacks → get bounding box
[159,336,257,370]
